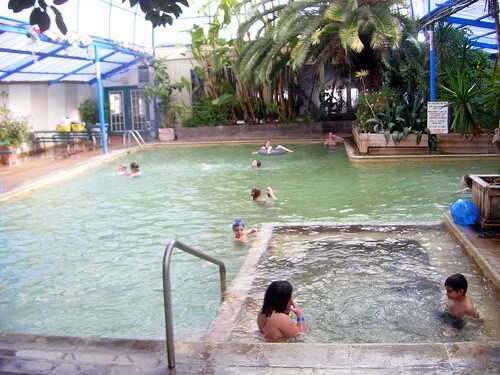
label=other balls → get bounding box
[450,198,478,226]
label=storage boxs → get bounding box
[55,124,85,132]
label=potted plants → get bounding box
[0,90,36,165]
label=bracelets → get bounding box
[296,317,305,322]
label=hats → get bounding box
[131,162,138,168]
[233,219,244,230]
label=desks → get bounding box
[32,130,111,150]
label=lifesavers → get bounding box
[258,149,285,155]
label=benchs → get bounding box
[32,136,88,153]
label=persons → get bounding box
[251,185,277,201]
[444,273,483,324]
[232,219,260,244]
[257,280,306,343]
[106,163,127,177]
[129,162,142,179]
[446,174,471,193]
[324,132,344,146]
[252,140,292,154]
[249,159,263,170]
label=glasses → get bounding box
[447,289,455,293]
